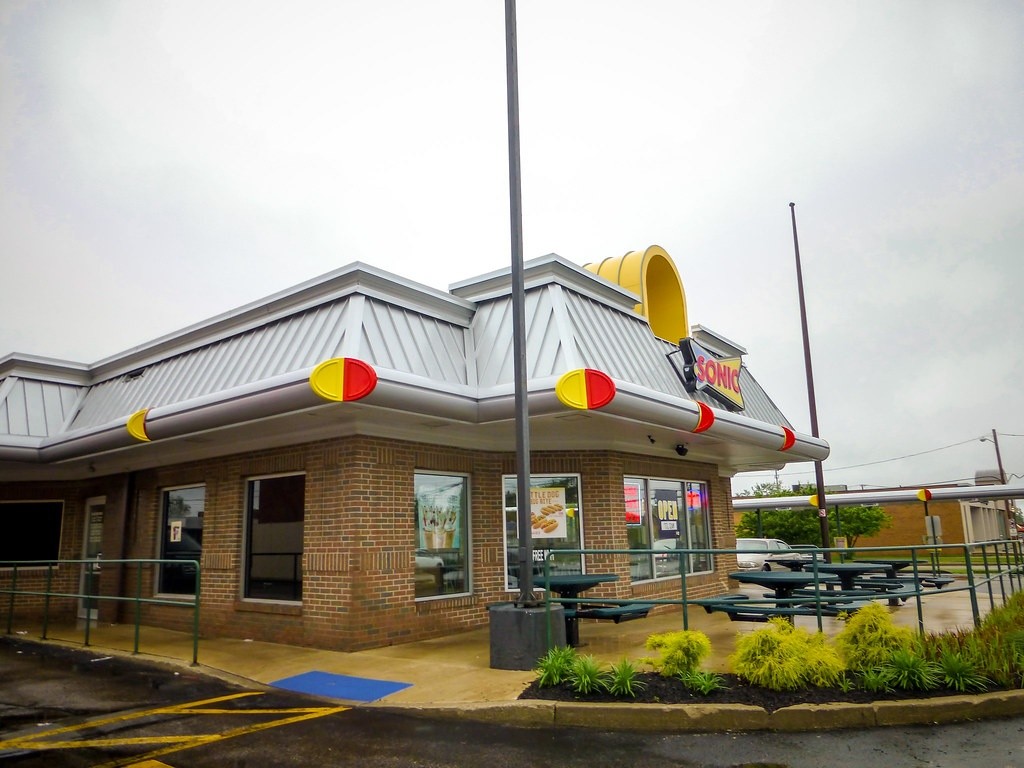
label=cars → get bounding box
[1015,523,1024,538]
[788,544,825,565]
[625,533,707,580]
[414,547,466,594]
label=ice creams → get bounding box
[422,504,456,550]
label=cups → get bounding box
[422,528,456,549]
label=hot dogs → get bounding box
[530,512,558,533]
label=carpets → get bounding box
[269,670,413,701]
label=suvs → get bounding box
[735,538,804,573]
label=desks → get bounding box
[534,575,620,647]
[727,559,927,631]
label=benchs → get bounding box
[695,575,955,622]
[485,598,655,624]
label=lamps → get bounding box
[675,443,689,456]
